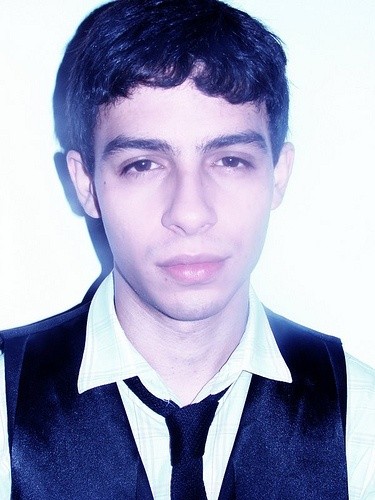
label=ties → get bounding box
[123,376,233,500]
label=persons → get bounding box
[0,0,375,500]
